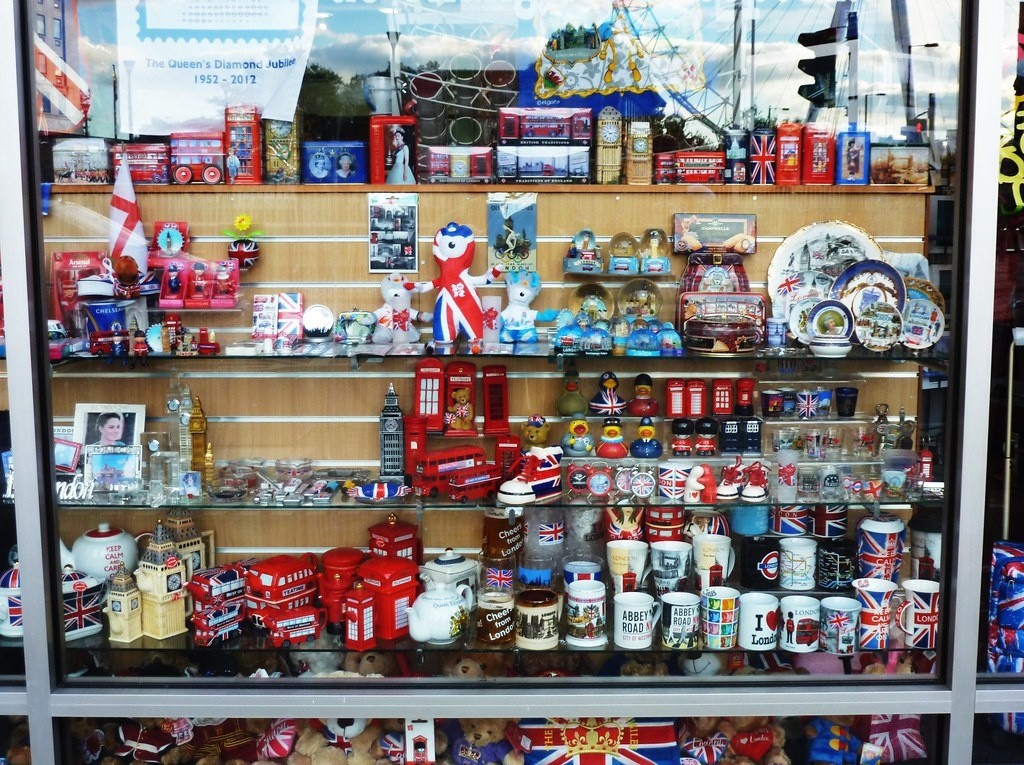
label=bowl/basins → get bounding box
[809,335,851,357]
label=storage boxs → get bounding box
[51,107,593,186]
[674,213,757,255]
[654,113,929,186]
[157,258,241,309]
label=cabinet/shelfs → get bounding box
[0,1,1024,765]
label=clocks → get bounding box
[596,106,653,185]
[262,104,305,183]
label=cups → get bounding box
[759,385,926,504]
[474,505,941,650]
[766,319,787,350]
[660,464,693,497]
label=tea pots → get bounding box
[0,523,154,641]
[418,547,482,611]
[405,582,473,645]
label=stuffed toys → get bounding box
[68,635,916,765]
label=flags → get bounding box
[110,154,148,274]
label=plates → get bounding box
[768,220,946,350]
[347,483,413,502]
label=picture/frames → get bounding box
[53,402,145,493]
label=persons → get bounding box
[216,265,231,294]
[91,412,126,445]
[386,127,416,185]
[129,330,149,369]
[336,155,355,179]
[105,331,126,366]
[167,265,182,294]
[226,148,240,185]
[192,263,205,293]
